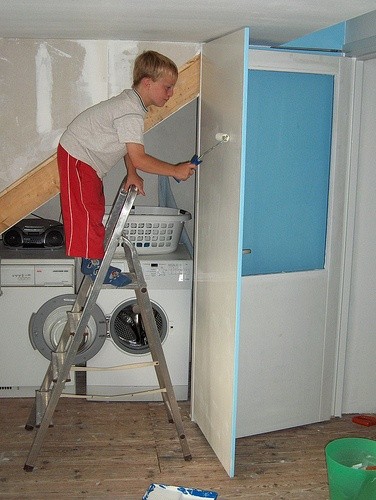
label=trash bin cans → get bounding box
[324,436,376,500]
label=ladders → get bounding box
[23,174,192,473]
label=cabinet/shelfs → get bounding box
[0,247,76,397]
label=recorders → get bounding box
[2,213,65,249]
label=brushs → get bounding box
[172,132,230,183]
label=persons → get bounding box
[57,50,196,286]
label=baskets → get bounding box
[102,205,191,254]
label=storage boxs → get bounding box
[102,206,192,255]
